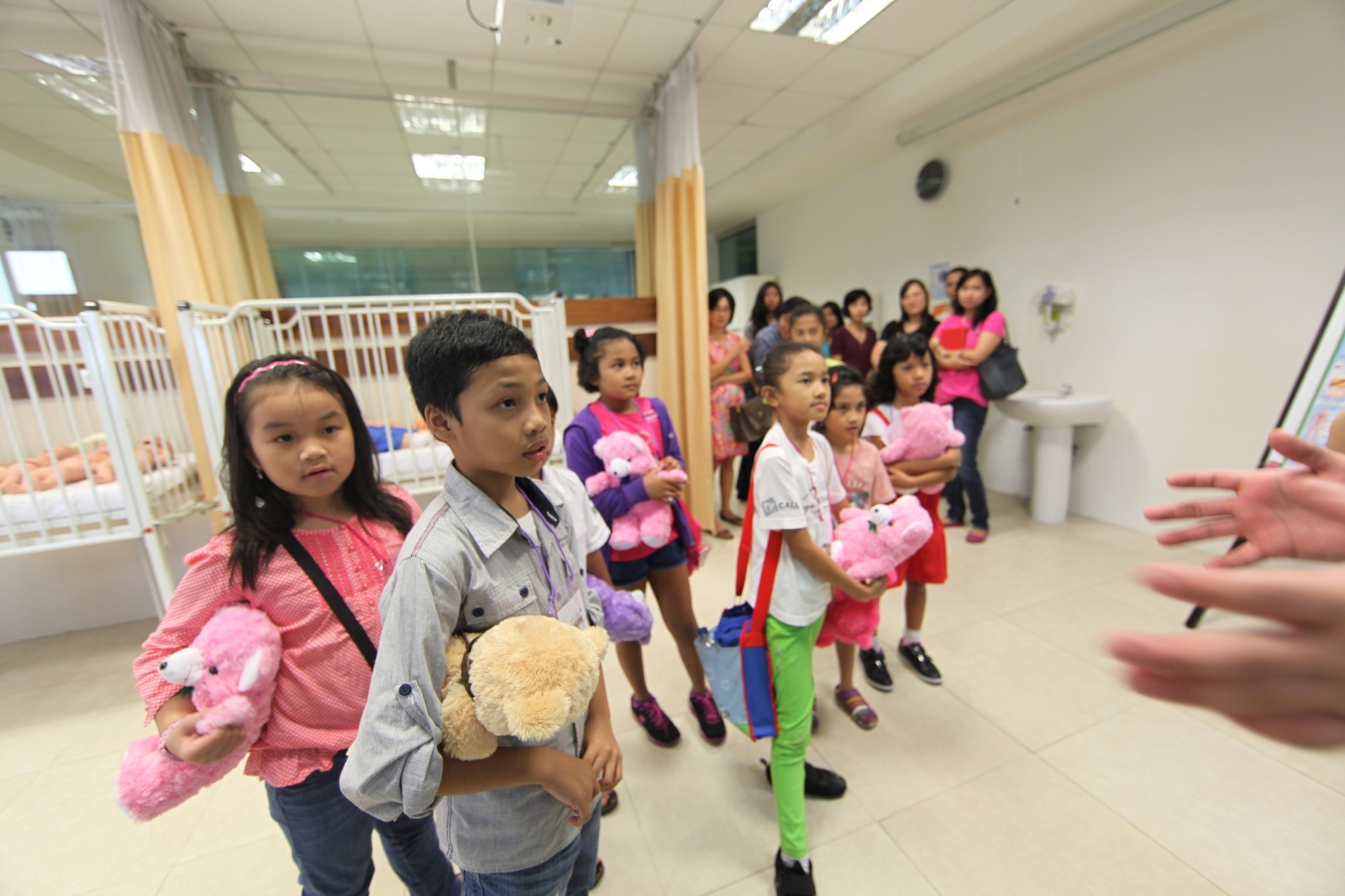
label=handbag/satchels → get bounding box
[977,313,1030,402]
[730,372,776,446]
[688,599,780,742]
[862,320,904,402]
[677,494,711,580]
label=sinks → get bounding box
[993,391,1112,426]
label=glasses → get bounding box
[710,306,734,316]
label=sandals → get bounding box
[720,508,744,527]
[706,526,733,540]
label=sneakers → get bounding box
[629,694,683,748]
[897,638,944,687]
[773,844,819,896]
[834,683,879,732]
[859,647,894,694]
[759,751,848,799]
[966,526,992,543]
[939,517,963,527]
[687,686,729,747]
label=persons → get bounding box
[133,352,463,896]
[704,267,1007,543]
[859,334,962,695]
[807,358,898,736]
[738,341,885,896]
[563,326,726,747]
[1102,428,1345,751]
[529,383,620,891]
[334,308,625,895]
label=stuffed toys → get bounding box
[832,492,933,583]
[441,614,610,762]
[584,430,689,549]
[876,401,966,494]
[585,571,654,646]
[119,598,281,822]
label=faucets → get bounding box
[1062,384,1074,395]
